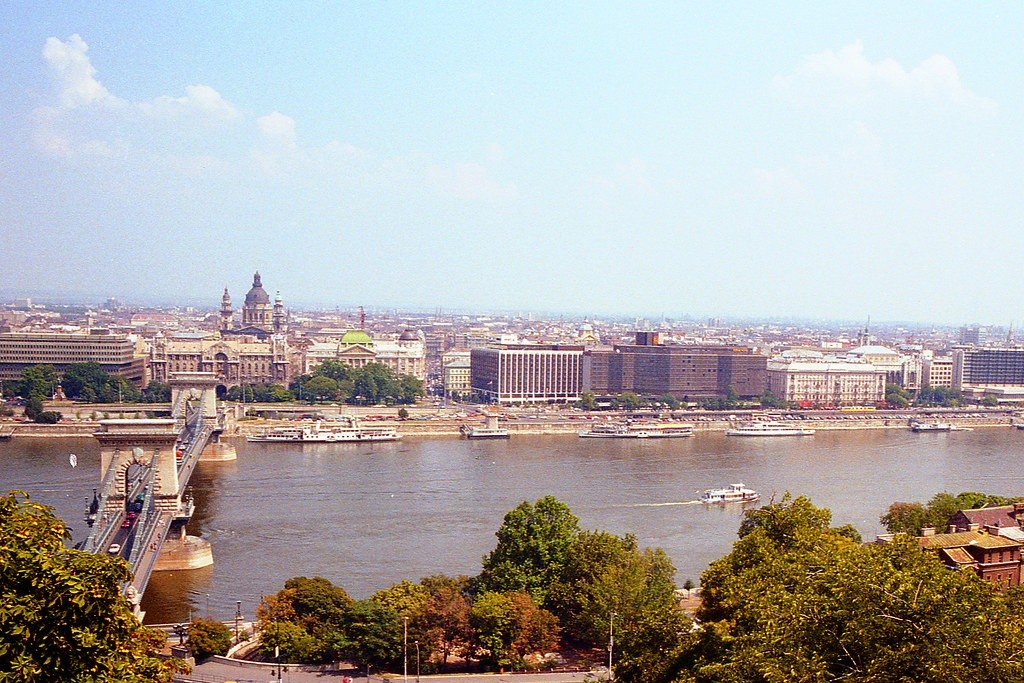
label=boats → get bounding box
[460,412,510,440]
[578,419,696,439]
[68,452,78,468]
[1012,423,1024,430]
[699,483,761,506]
[725,420,817,437]
[245,421,407,443]
[910,420,956,432]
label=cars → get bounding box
[476,406,1024,421]
[260,413,471,421]
[175,436,192,467]
[108,482,150,555]
